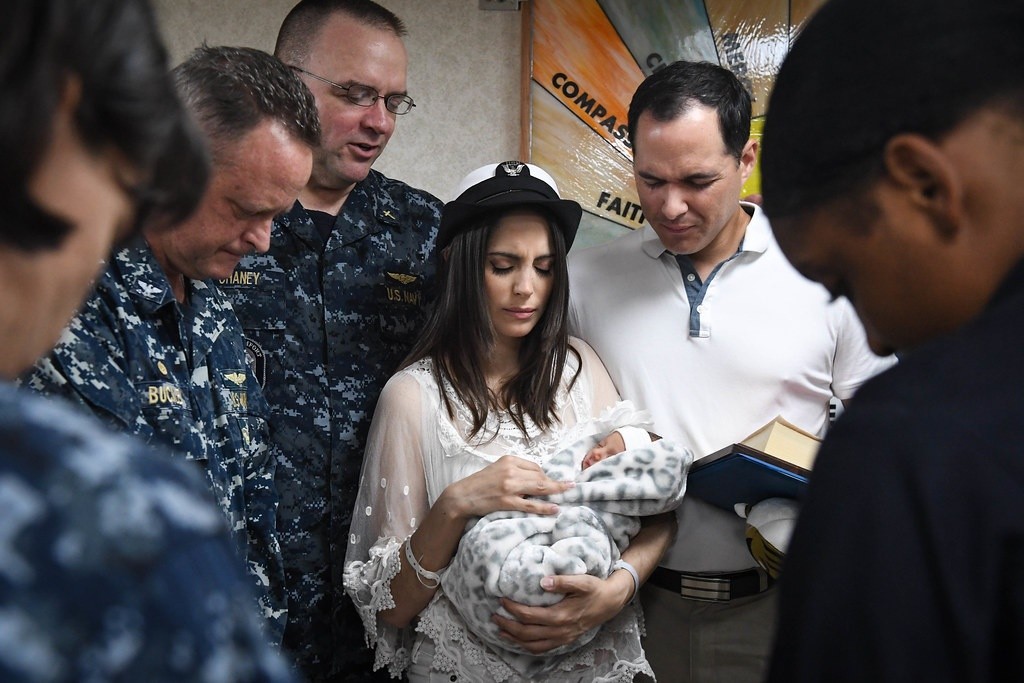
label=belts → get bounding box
[650,566,777,603]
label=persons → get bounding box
[343,158,689,683]
[234,0,445,683]
[761,0,1022,683]
[567,58,895,683]
[0,0,322,683]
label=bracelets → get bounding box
[403,535,444,586]
[613,558,639,608]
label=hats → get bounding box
[436,160,582,262]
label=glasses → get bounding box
[287,65,416,115]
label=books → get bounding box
[739,414,823,471]
